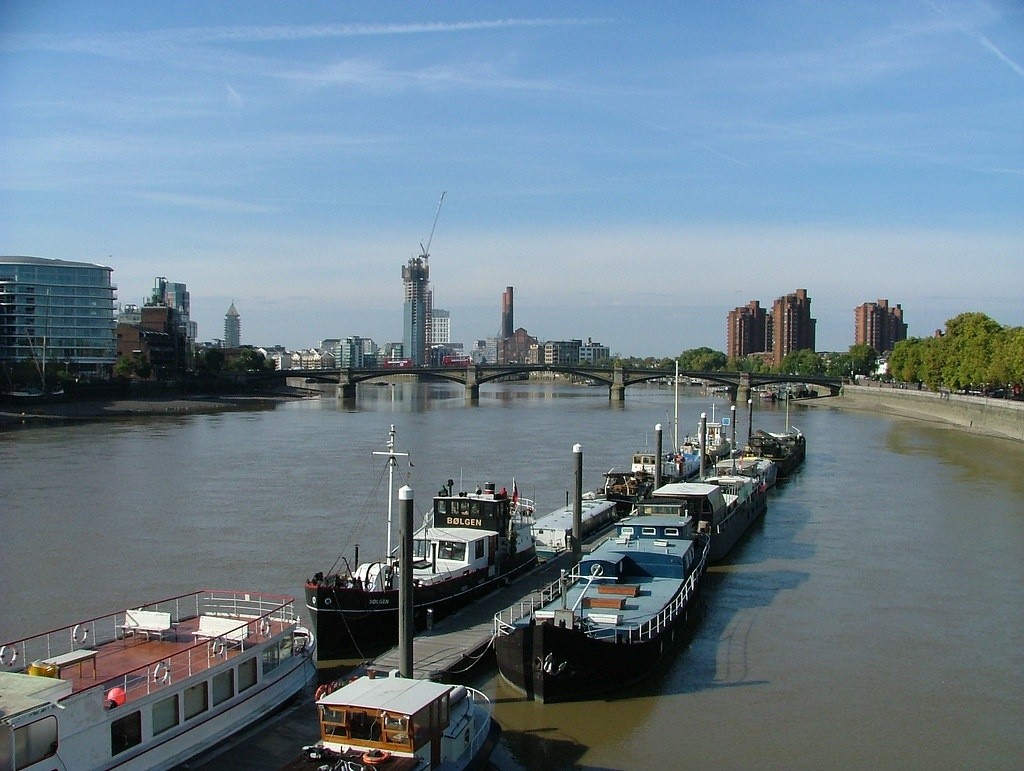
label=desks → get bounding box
[41,649,99,680]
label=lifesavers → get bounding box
[154,662,170,685]
[260,617,272,638]
[73,622,89,646]
[363,751,388,763]
[0,643,18,666]
[213,636,226,659]
[314,684,327,701]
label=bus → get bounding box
[442,354,474,366]
[384,357,413,368]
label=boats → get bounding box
[491,496,711,705]
[711,455,778,491]
[530,490,636,559]
[595,467,670,517]
[0,589,319,771]
[303,424,540,663]
[706,380,819,403]
[613,473,768,566]
[684,402,731,466]
[284,669,493,771]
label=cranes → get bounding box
[419,190,449,281]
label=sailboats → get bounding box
[630,359,704,485]
[734,393,808,479]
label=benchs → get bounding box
[121,609,171,644]
[192,616,255,652]
[171,614,181,643]
